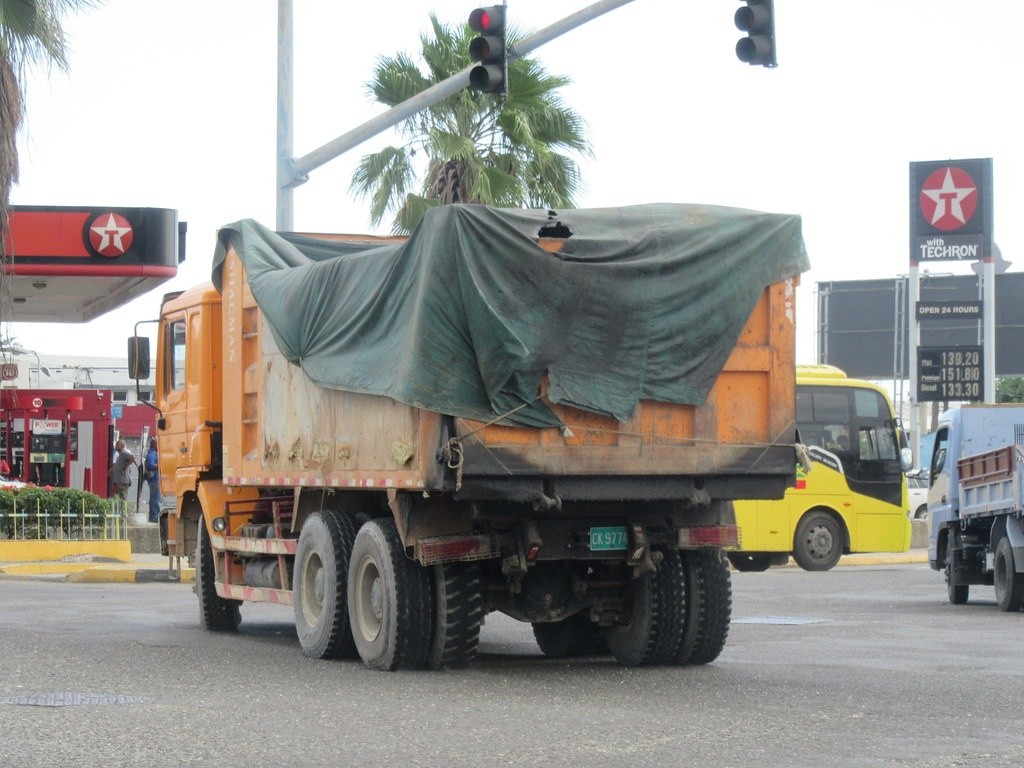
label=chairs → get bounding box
[821,430,835,448]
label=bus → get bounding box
[718,363,911,571]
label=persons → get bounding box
[145,437,164,521]
[111,440,135,500]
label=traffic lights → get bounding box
[469,1,508,105]
[735,0,778,69]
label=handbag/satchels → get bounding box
[144,470,155,479]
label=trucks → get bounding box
[126,204,799,672]
[900,403,1024,611]
